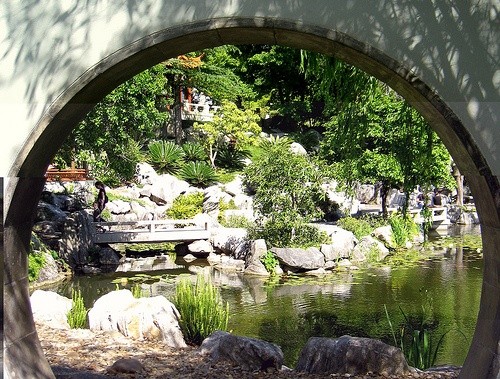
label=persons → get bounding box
[95,180,112,232]
[416,193,428,208]
[431,190,444,208]
[92,202,107,234]
